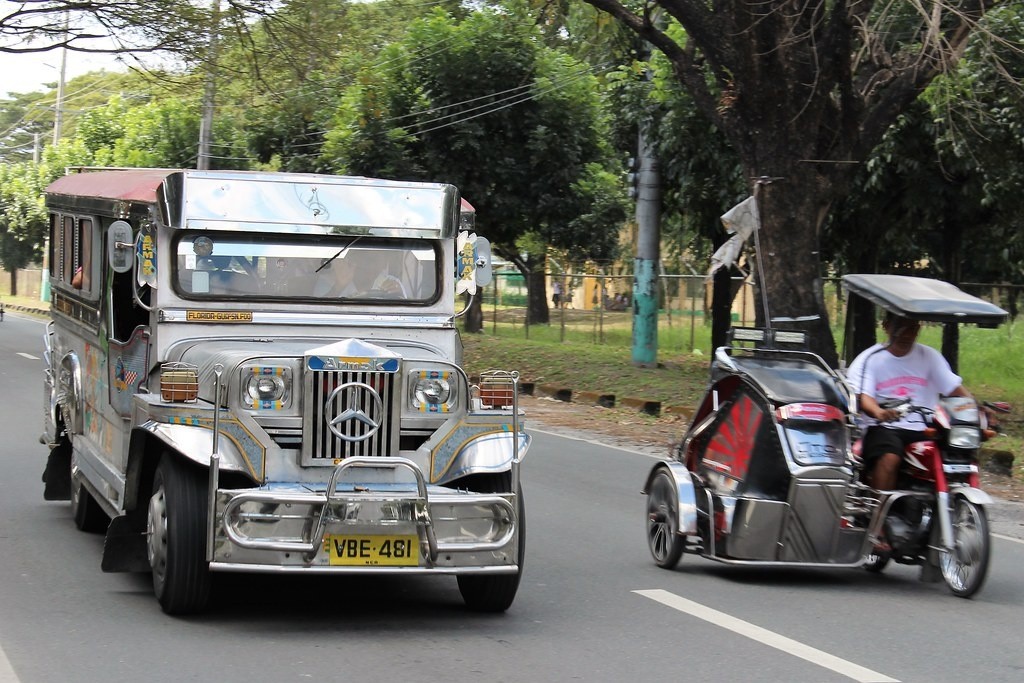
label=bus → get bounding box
[39,165,528,613]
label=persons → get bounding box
[312,252,407,298]
[846,311,980,551]
[551,278,560,308]
[72,266,83,288]
[558,282,574,309]
[592,284,628,312]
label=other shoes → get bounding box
[874,536,892,552]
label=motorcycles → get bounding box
[641,273,1009,600]
